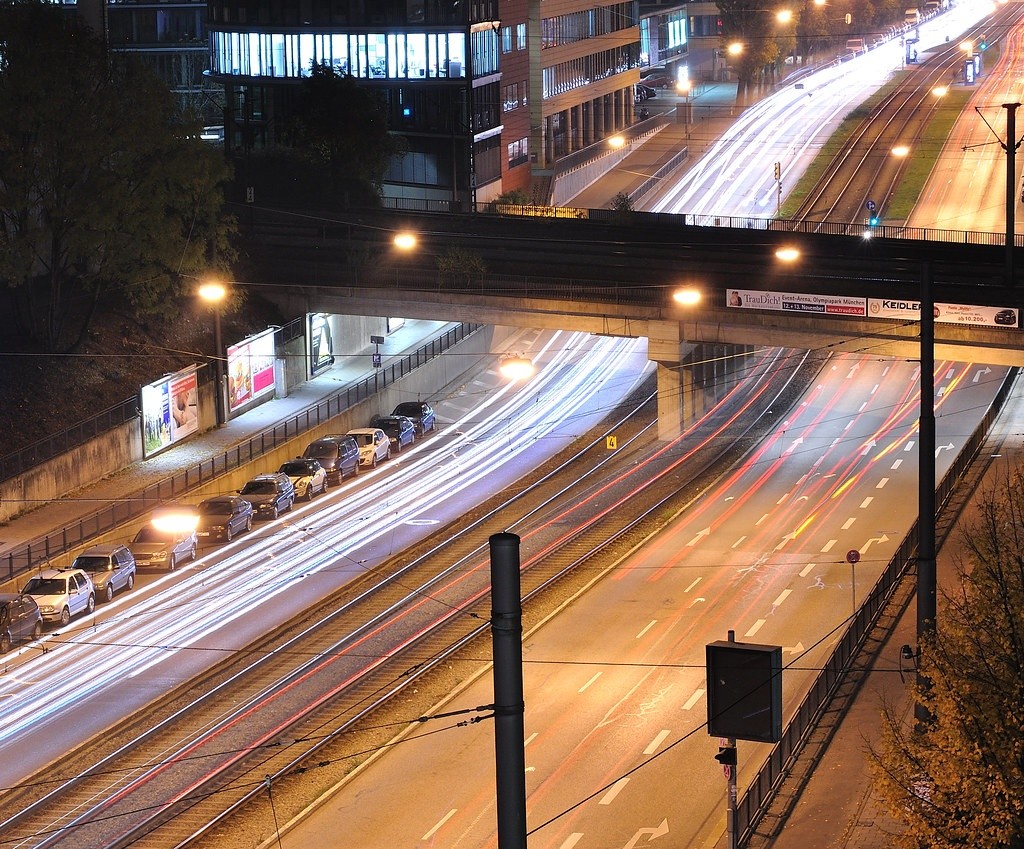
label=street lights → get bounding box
[676,79,694,141]
[197,284,227,429]
[672,287,699,435]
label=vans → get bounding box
[295,433,360,487]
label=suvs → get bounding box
[275,459,329,502]
[236,471,296,520]
[68,544,136,602]
[16,566,95,627]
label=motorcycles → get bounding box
[639,105,649,121]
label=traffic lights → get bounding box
[980,44,986,50]
[870,209,878,227]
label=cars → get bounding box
[0,593,43,654]
[846,38,864,54]
[639,71,675,90]
[344,428,391,469]
[127,521,199,572]
[390,401,436,438]
[191,493,254,543]
[869,0,952,45]
[367,415,416,452]
[635,84,657,104]
